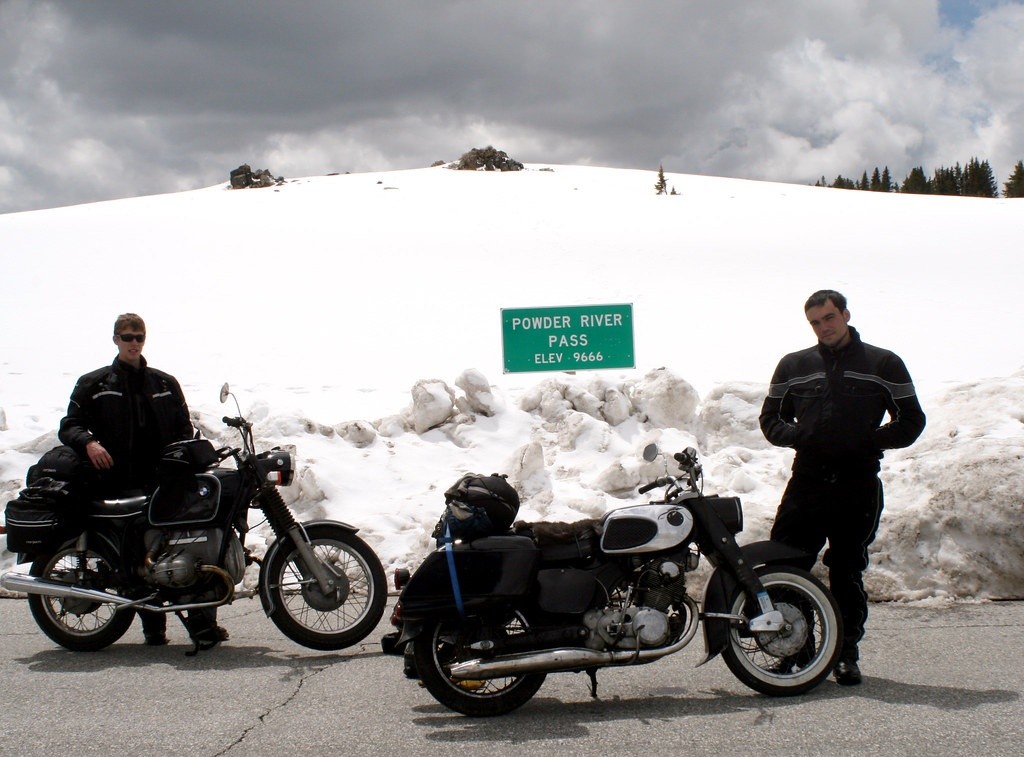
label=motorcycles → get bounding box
[0,382,388,652]
[381,443,843,718]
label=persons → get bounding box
[57,312,229,651]
[758,289,927,687]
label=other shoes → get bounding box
[766,649,816,676]
[835,660,863,685]
[143,630,168,647]
[188,626,230,641]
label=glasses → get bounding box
[115,332,146,343]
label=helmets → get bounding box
[444,473,518,536]
[159,429,221,473]
